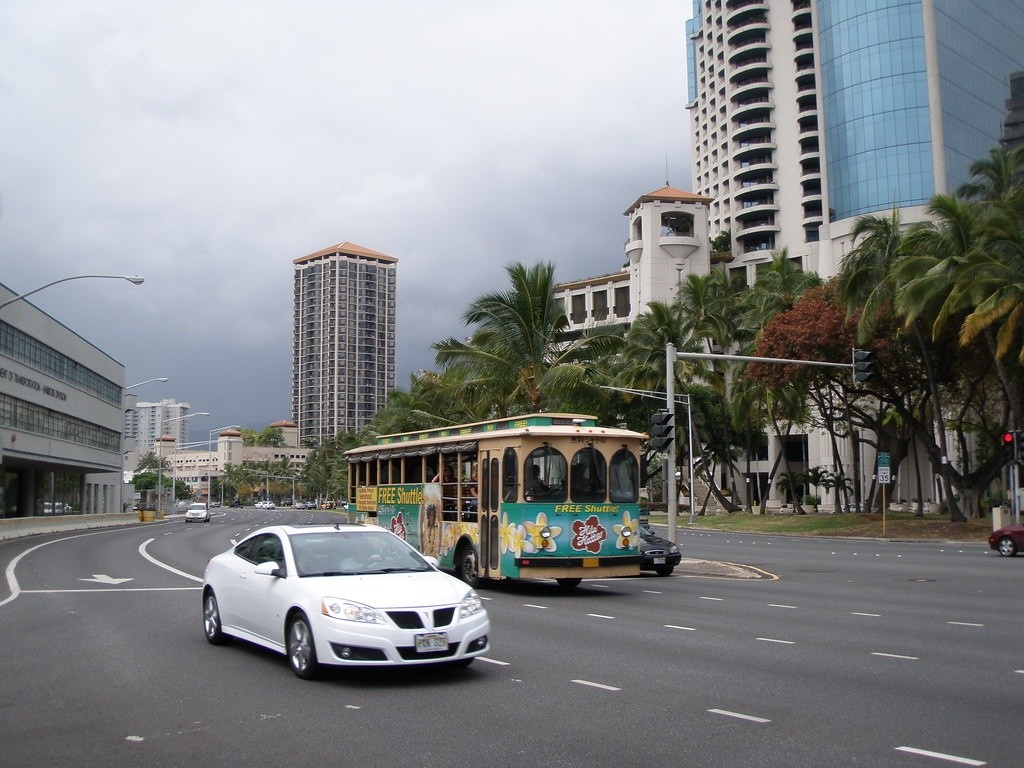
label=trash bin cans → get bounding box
[142,508,157,522]
[992,508,1011,531]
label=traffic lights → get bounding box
[855,350,876,385]
[651,411,675,451]
[1016,430,1024,464]
[1002,434,1014,445]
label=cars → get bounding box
[229,500,244,509]
[254,500,276,511]
[210,502,221,508]
[45,502,72,515]
[639,522,683,579]
[988,522,1024,558]
[200,520,492,680]
[280,497,349,510]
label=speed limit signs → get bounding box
[879,467,891,484]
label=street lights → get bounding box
[157,411,211,512]
[207,425,241,510]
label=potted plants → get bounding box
[137,508,157,523]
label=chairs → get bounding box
[302,539,370,574]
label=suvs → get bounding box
[184,502,211,522]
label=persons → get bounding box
[420,498,442,555]
[340,548,392,570]
[525,465,546,492]
[432,465,457,503]
[468,464,478,508]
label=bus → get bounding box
[342,412,651,589]
